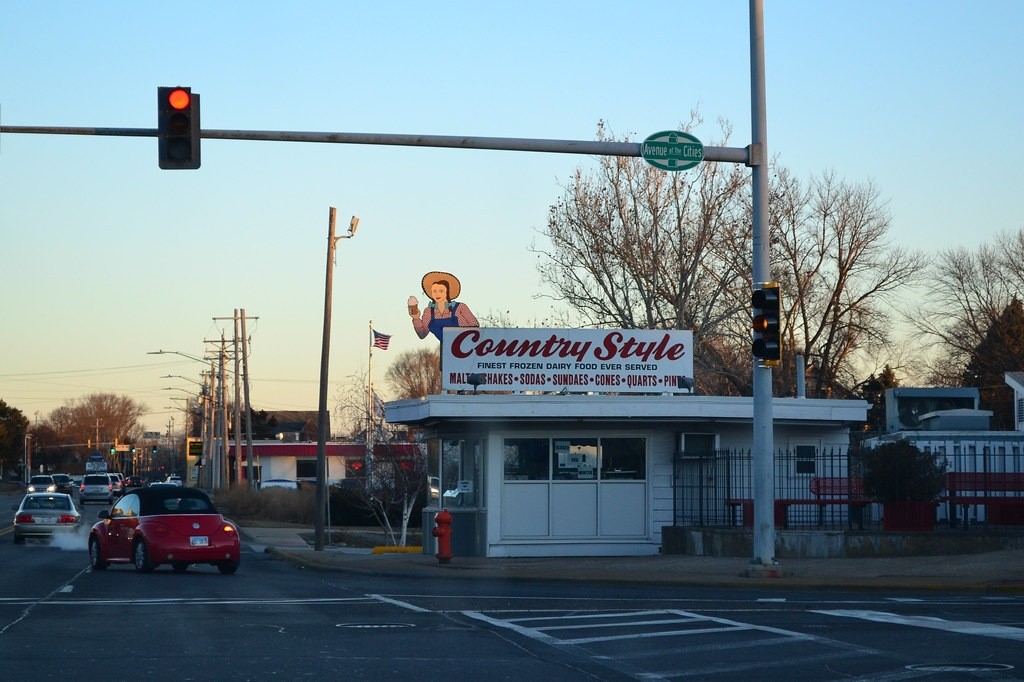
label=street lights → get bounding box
[314,206,358,552]
[149,351,254,490]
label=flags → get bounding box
[372,330,392,350]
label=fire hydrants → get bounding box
[432,508,454,563]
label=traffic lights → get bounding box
[157,86,197,169]
[752,287,780,362]
[111,445,114,453]
[132,445,136,453]
[153,445,157,453]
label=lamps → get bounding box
[677,376,697,396]
[468,372,487,396]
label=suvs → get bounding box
[79,473,113,504]
[165,477,182,486]
[26,475,57,494]
[51,474,73,496]
[128,476,143,487]
[105,473,126,496]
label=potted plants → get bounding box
[866,440,955,533]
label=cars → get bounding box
[150,483,179,489]
[14,492,82,547]
[88,488,240,577]
[429,477,463,506]
